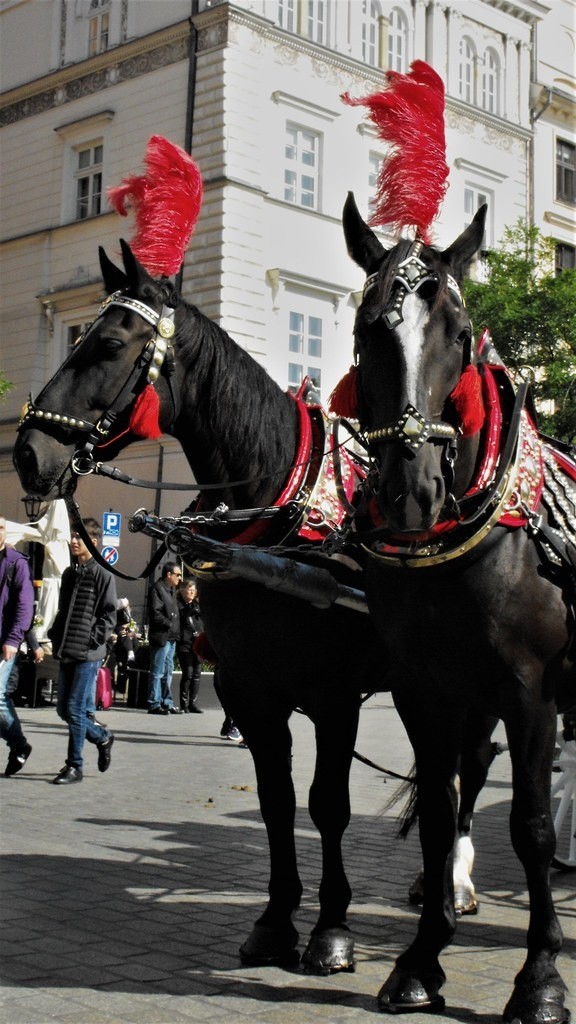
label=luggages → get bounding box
[94,667,112,711]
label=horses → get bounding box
[12,239,576,975]
[344,192,576,1024]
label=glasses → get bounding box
[169,572,183,577]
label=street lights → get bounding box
[18,490,45,707]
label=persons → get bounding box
[0,514,35,777]
[219,716,242,741]
[148,562,204,717]
[5,627,44,720]
[46,517,117,785]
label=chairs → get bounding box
[33,656,60,709]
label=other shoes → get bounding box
[191,703,204,714]
[164,704,184,714]
[227,725,244,742]
[181,707,190,714]
[5,742,32,777]
[149,706,168,714]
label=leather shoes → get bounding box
[53,763,82,784]
[96,735,115,772]
